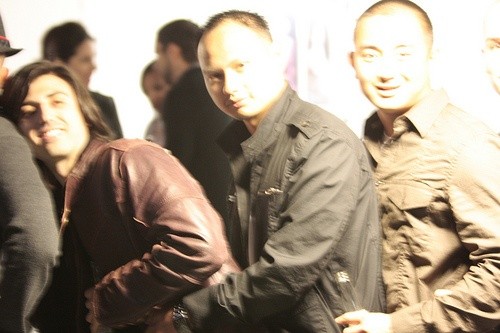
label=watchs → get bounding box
[171,302,192,333]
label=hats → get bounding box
[0,13,24,57]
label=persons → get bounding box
[0,12,61,333]
[0,61,242,332]
[335,0,499,333]
[468,37,498,137]
[144,10,386,333]
[41,19,236,246]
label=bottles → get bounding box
[334,271,364,311]
